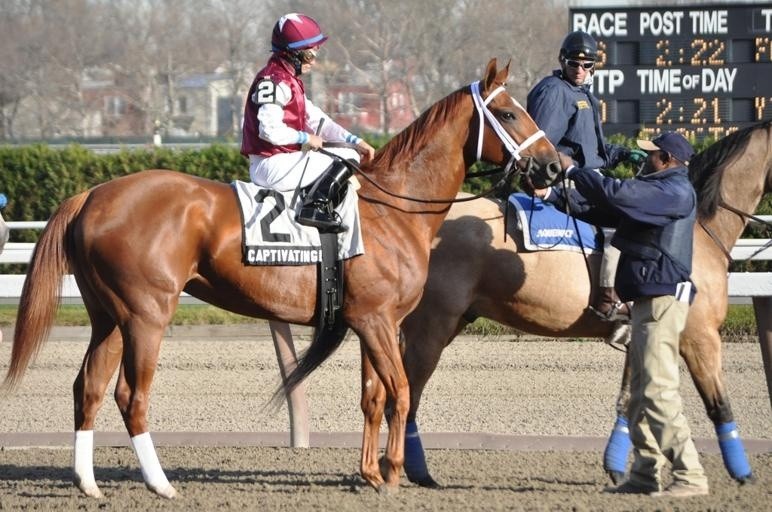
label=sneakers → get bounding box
[603,479,664,496]
[649,480,709,498]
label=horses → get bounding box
[256,120,772,489]
[0,58,563,499]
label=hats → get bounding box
[636,132,696,168]
[271,13,329,76]
[560,31,598,60]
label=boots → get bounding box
[596,286,633,322]
[296,159,353,234]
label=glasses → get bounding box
[563,58,596,72]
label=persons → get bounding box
[526,31,650,322]
[152,118,163,146]
[241,12,376,228]
[517,131,711,498]
